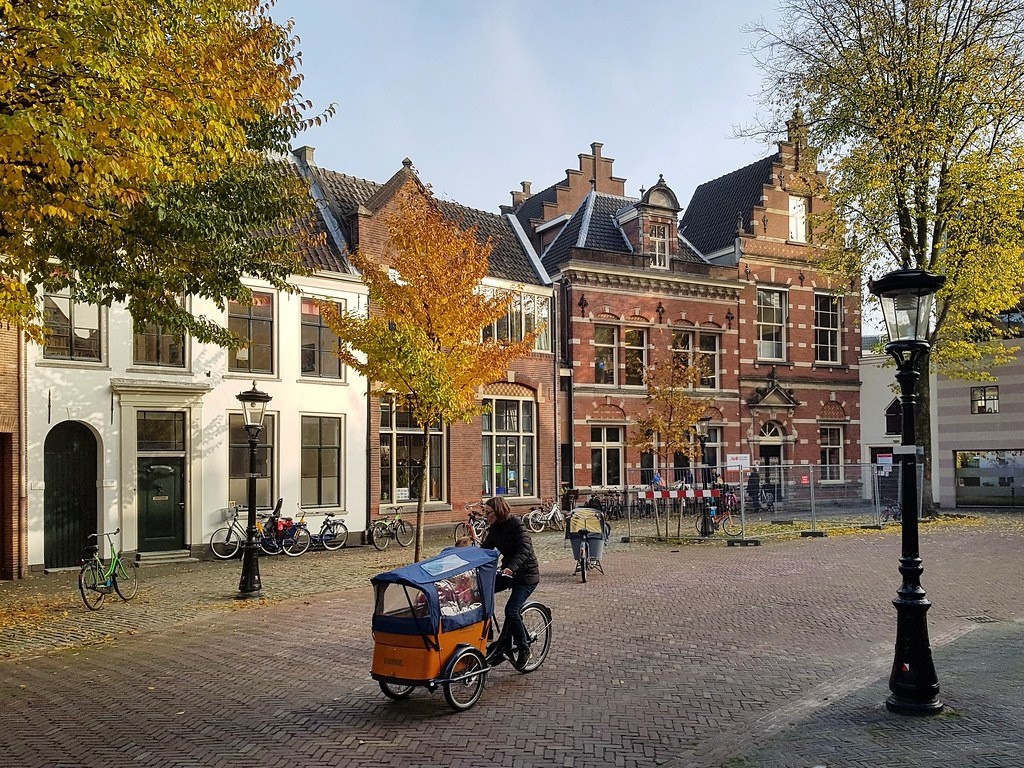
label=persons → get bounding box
[589,494,603,514]
[747,468,762,513]
[712,469,723,484]
[480,495,540,669]
[683,469,693,484]
[999,460,1014,487]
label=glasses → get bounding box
[486,510,495,515]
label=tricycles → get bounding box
[369,546,554,712]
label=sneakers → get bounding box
[516,648,533,670]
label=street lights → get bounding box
[235,379,273,600]
[867,247,946,715]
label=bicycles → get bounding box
[521,506,536,532]
[600,486,623,520]
[696,494,742,536]
[454,501,490,545]
[528,495,564,533]
[371,506,415,551]
[563,510,604,582]
[210,504,283,560]
[293,502,348,557]
[78,527,138,611]
[240,517,311,561]
